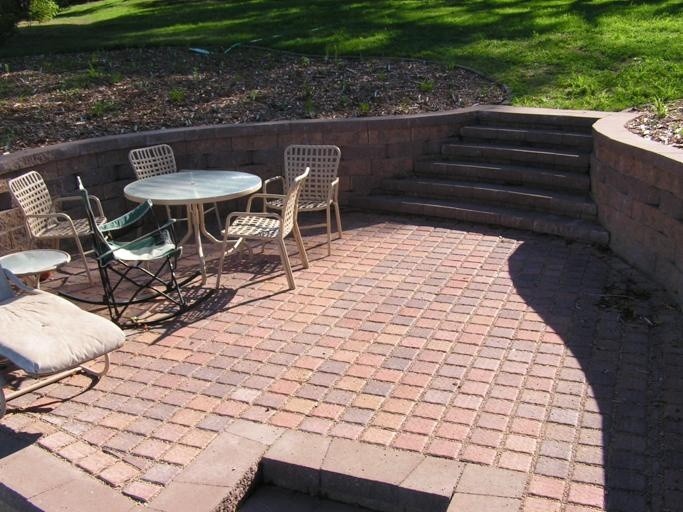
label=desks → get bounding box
[0,248,71,290]
[124,169,262,284]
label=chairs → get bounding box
[0,265,125,419]
[128,144,223,244]
[8,172,105,290]
[260,145,342,256]
[215,165,310,293]
[76,176,214,331]
